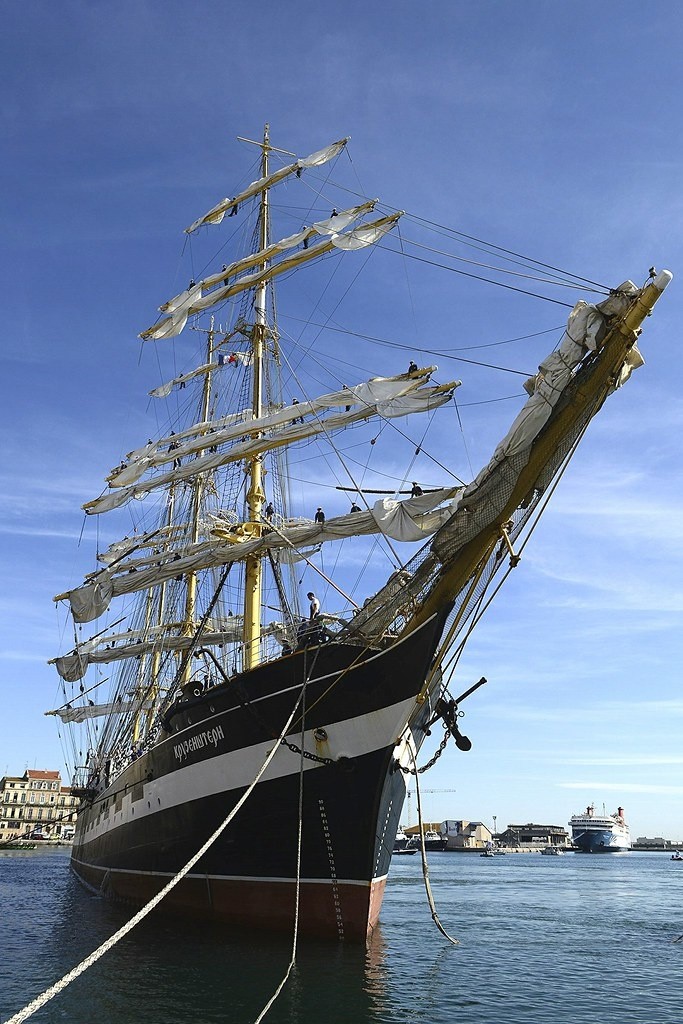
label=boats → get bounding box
[489,851,505,855]
[568,802,631,853]
[392,849,418,856]
[670,855,683,860]
[539,850,558,855]
[0,844,37,850]
[481,853,494,857]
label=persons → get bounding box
[307,592,321,646]
[331,208,338,218]
[179,374,186,389]
[168,440,182,471]
[408,361,418,380]
[123,530,148,541]
[315,508,325,524]
[147,438,153,444]
[121,461,127,470]
[410,482,424,499]
[265,502,275,522]
[296,167,303,178]
[156,550,162,566]
[222,264,229,286]
[209,427,217,453]
[131,745,143,762]
[350,502,363,513]
[129,566,137,573]
[281,638,292,656]
[65,608,264,711]
[292,398,305,425]
[296,618,311,643]
[302,225,309,250]
[228,197,238,217]
[190,279,196,290]
[170,431,176,436]
[343,384,351,412]
[174,553,181,561]
[6,832,73,841]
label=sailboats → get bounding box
[42,123,676,932]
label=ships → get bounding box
[391,821,448,851]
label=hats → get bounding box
[352,502,356,504]
[317,507,322,510]
